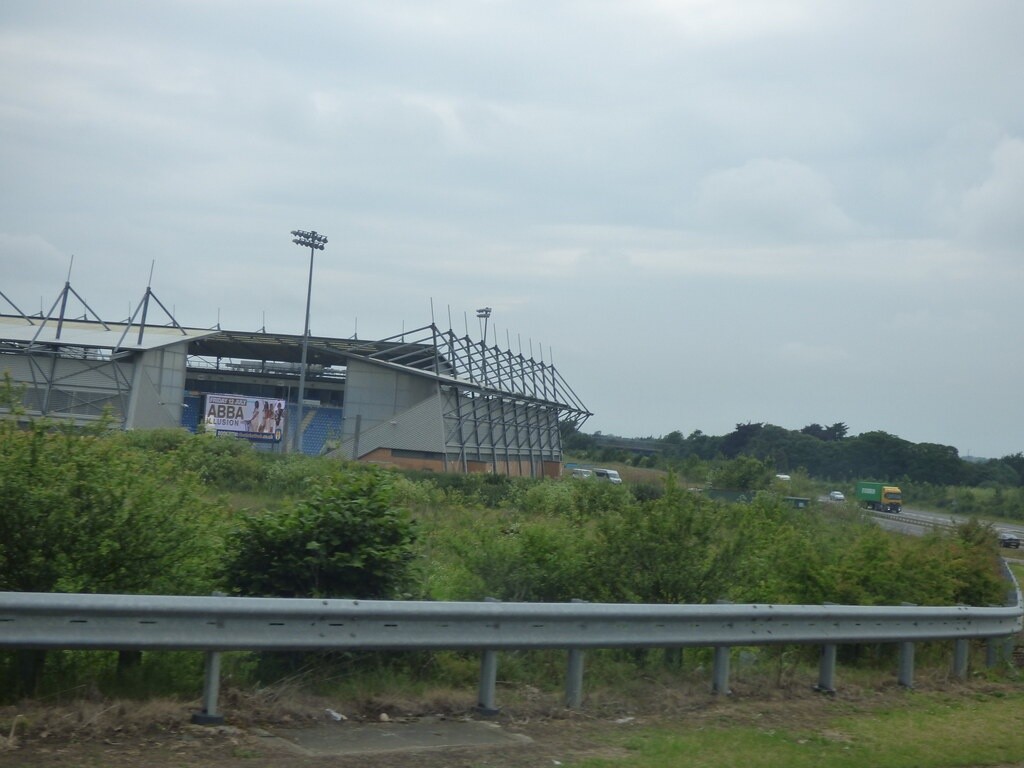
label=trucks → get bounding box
[855,482,902,513]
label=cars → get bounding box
[830,492,844,501]
[998,534,1020,549]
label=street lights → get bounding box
[291,229,329,455]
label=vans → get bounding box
[573,469,592,479]
[592,468,622,484]
